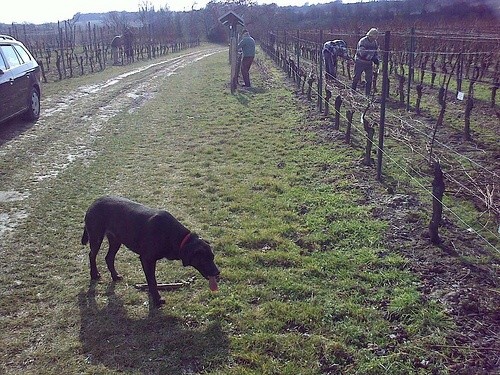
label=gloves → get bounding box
[372,57,379,65]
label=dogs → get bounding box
[81,196,220,306]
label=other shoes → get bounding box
[114,61,121,64]
[242,83,250,86]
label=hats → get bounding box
[241,29,249,35]
[366,27,379,36]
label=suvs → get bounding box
[0,34,42,126]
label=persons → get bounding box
[111,35,123,64]
[237,29,255,87]
[322,39,354,79]
[121,28,134,57]
[351,28,379,96]
